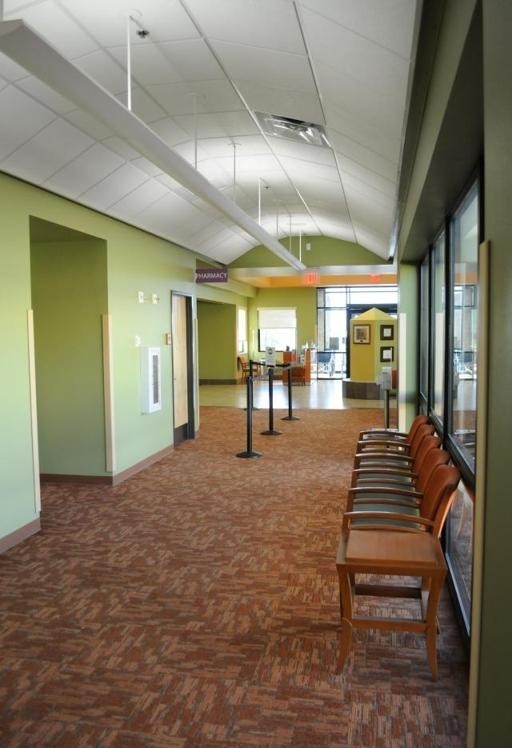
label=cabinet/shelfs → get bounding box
[282,350,316,384]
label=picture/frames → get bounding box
[380,346,395,363]
[380,324,394,340]
[352,323,371,345]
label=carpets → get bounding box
[1,402,468,747]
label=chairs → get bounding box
[317,352,332,375]
[237,353,262,384]
[334,414,462,685]
[291,365,307,386]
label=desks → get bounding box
[322,351,347,376]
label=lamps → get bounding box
[0,3,308,278]
